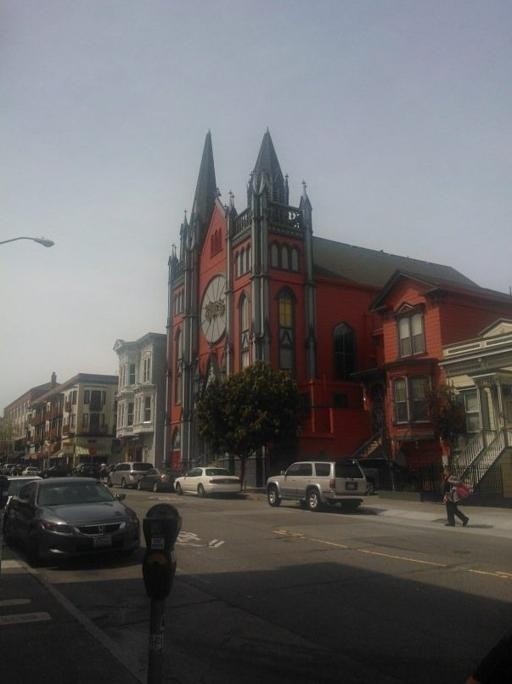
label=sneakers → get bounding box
[444,517,469,527]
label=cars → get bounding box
[173,466,241,497]
[138,468,182,497]
[0,461,153,567]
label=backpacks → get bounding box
[448,474,470,504]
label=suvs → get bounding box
[266,460,384,512]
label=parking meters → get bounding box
[142,504,181,683]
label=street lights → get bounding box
[0,237,54,248]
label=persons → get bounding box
[100,462,107,474]
[440,465,469,526]
